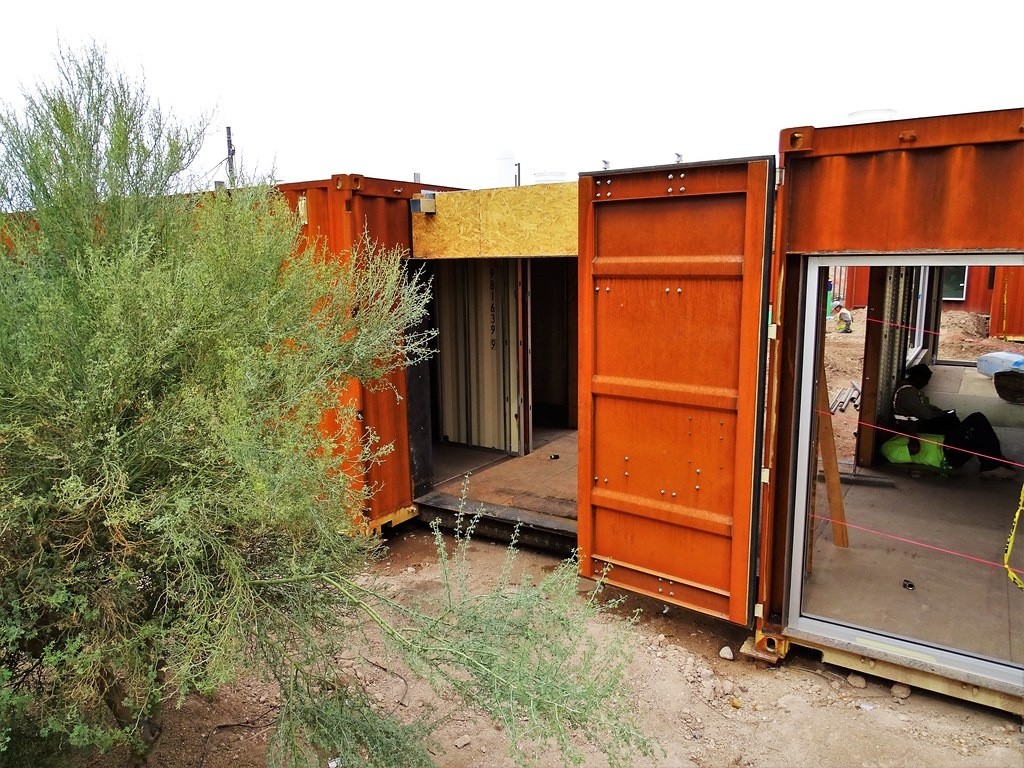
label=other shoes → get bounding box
[978,467,1017,480]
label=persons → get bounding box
[832,302,853,333]
[875,364,1017,479]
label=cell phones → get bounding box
[947,409,956,414]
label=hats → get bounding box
[831,302,840,310]
